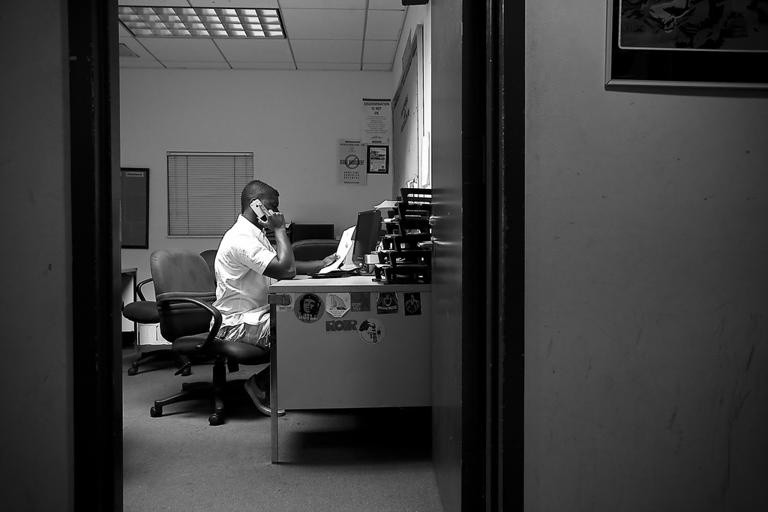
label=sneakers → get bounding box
[243,373,286,416]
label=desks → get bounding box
[269,272,431,463]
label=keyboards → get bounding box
[313,263,343,278]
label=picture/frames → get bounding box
[601,0,766,96]
[367,145,390,175]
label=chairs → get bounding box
[148,249,266,424]
[125,250,223,375]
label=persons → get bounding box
[299,294,320,318]
[210,179,297,418]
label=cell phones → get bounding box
[250,199,268,219]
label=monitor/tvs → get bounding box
[352,210,381,276]
